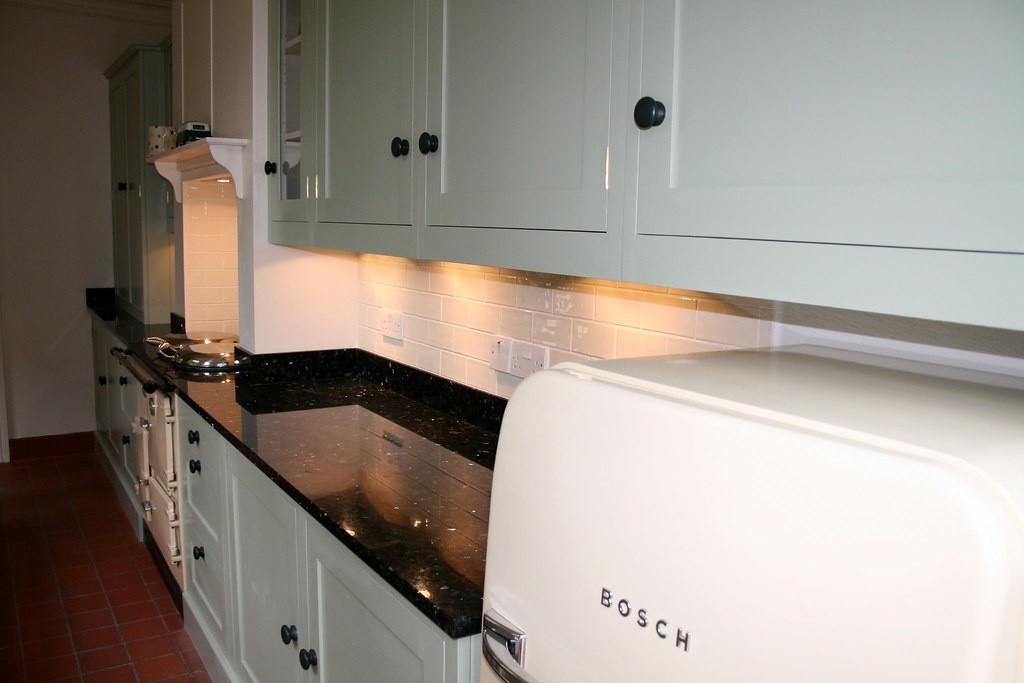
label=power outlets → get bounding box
[381,308,403,340]
[488,334,549,379]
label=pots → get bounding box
[146,331,239,346]
[158,342,252,370]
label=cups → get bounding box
[148,126,169,154]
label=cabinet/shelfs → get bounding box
[102,42,175,324]
[267,0,1024,332]
[90,312,139,497]
[175,396,483,683]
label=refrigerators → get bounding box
[478,349,1024,683]
